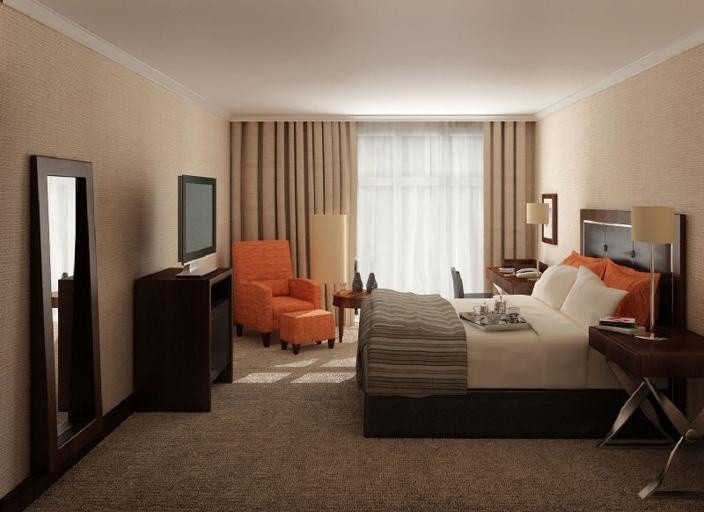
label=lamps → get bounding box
[526,202,549,270]
[310,214,348,312]
[629,204,676,335]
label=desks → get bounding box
[487,265,536,295]
[333,291,369,343]
[588,324,703,499]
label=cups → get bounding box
[473,296,521,326]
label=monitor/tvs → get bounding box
[177,173,217,263]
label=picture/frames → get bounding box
[541,192,558,245]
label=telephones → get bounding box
[514,267,542,279]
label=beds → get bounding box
[355,208,686,440]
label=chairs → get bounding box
[231,239,323,348]
[451,266,495,298]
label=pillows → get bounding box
[530,262,578,311]
[603,256,661,328]
[561,249,608,281]
[560,265,630,333]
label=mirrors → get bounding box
[29,154,106,471]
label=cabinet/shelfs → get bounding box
[58,277,75,412]
[132,267,234,412]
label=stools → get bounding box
[279,309,337,355]
